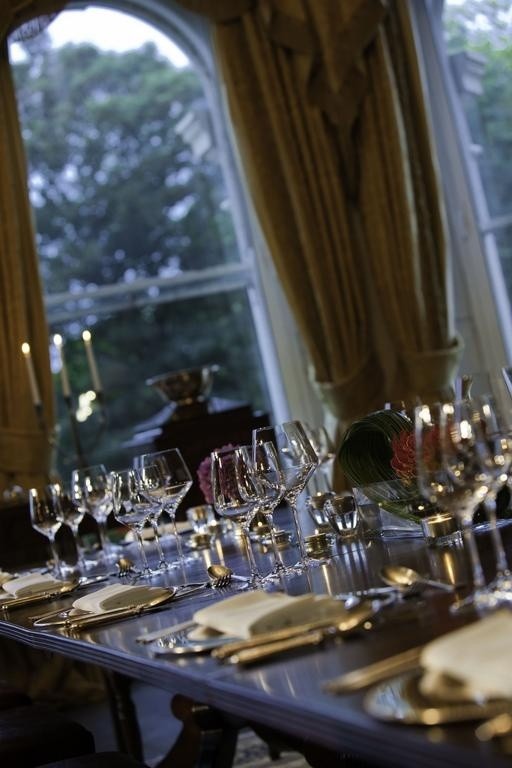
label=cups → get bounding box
[186,504,217,546]
[324,496,359,539]
[305,492,333,534]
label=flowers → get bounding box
[197,444,260,505]
[338,409,453,524]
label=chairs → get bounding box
[0,688,149,768]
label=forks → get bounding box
[181,574,232,589]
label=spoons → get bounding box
[61,587,176,630]
[378,561,511,609]
[207,564,276,586]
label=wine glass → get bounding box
[111,446,195,581]
[207,421,328,589]
[26,463,124,579]
[414,355,511,619]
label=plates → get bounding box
[363,669,511,725]
[31,586,177,628]
[151,591,396,655]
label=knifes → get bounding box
[230,599,393,664]
[72,606,172,629]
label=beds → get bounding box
[0,507,512,768]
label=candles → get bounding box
[53,333,70,397]
[83,330,102,392]
[21,342,41,404]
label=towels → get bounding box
[72,583,169,614]
[192,589,349,639]
[3,573,63,597]
[419,608,511,699]
[125,522,191,543]
